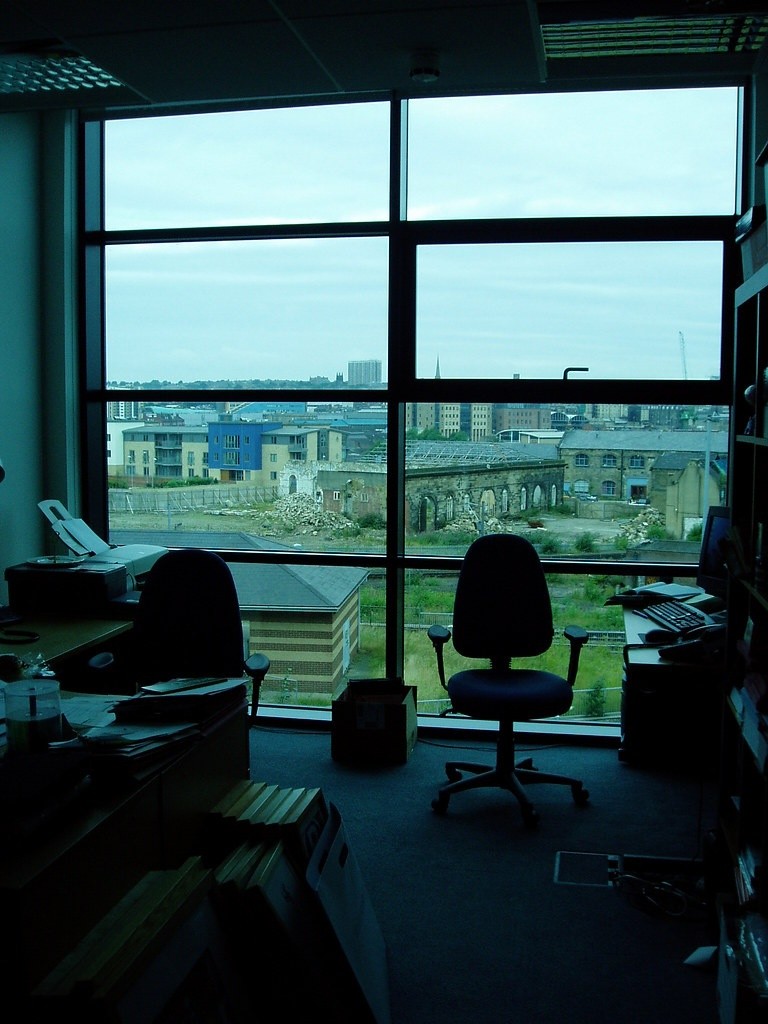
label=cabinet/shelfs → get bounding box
[717,261,768,1024]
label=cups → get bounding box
[3,678,63,755]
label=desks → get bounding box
[0,604,251,1024]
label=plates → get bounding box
[29,557,85,567]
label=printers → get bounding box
[38,499,169,593]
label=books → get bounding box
[82,672,249,761]
[603,581,713,605]
[720,808,768,908]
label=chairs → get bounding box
[429,532,594,821]
[87,550,272,729]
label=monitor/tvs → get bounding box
[696,505,733,601]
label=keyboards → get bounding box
[643,600,717,635]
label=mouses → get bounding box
[645,630,678,642]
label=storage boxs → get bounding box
[331,679,420,770]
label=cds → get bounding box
[7,707,62,745]
